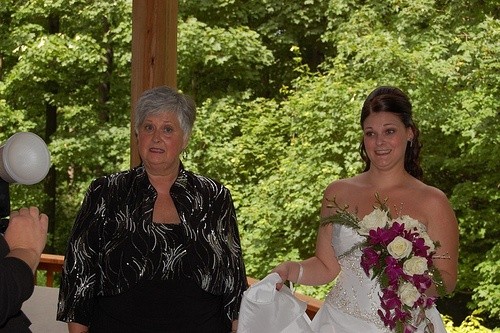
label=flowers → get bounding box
[314,192,454,333]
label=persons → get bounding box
[0,207,49,333]
[236,86,458,333]
[56,85,249,333]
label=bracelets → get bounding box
[294,261,303,287]
